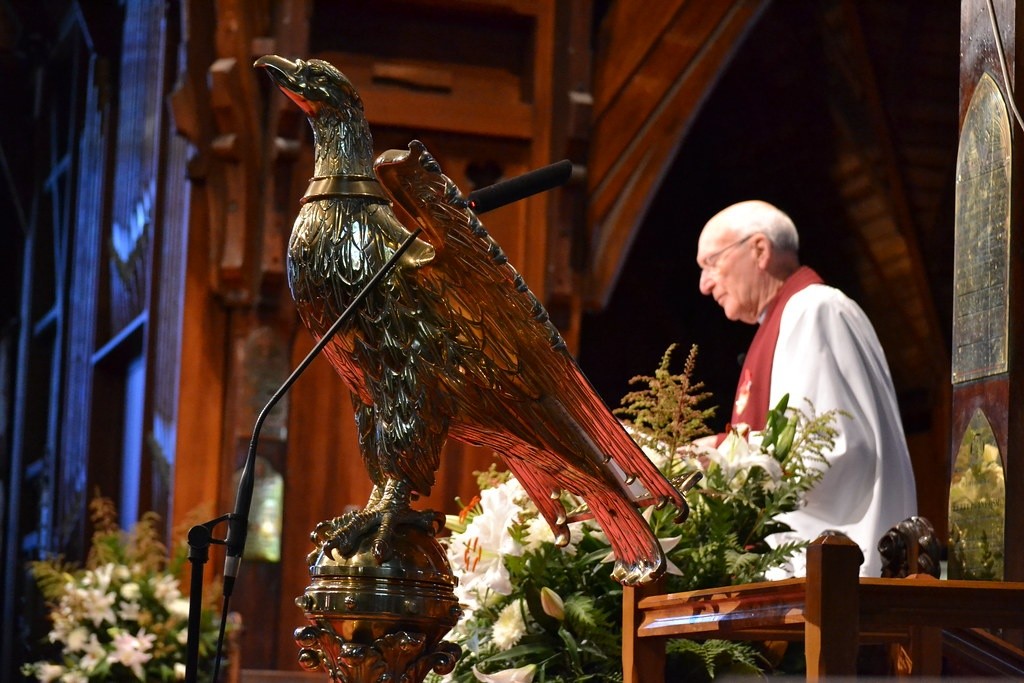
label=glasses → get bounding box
[706,232,774,265]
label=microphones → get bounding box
[222,159,573,593]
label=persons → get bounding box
[675,201,917,581]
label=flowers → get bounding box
[424,344,856,683]
[20,487,241,683]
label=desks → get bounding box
[622,530,1024,683]
